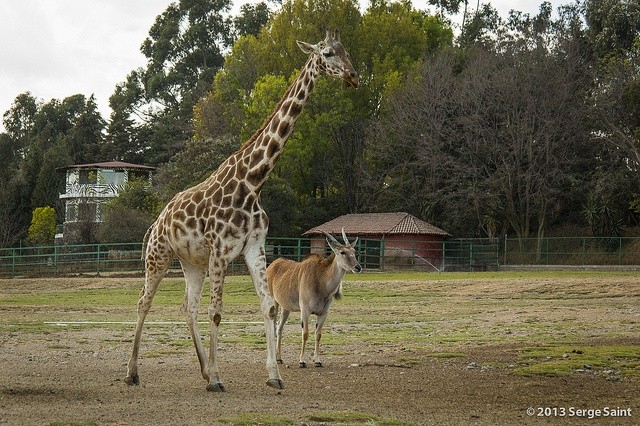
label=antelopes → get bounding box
[267,227,362,368]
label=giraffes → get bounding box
[124,27,362,392]
